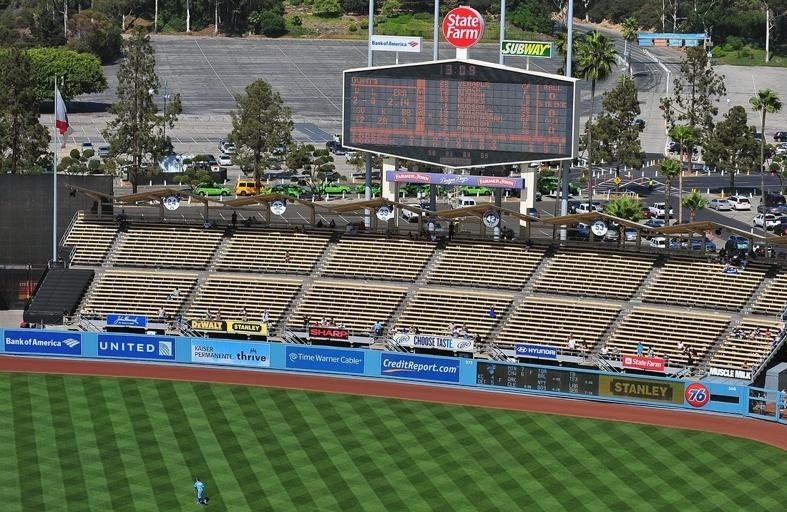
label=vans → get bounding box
[235,180,268,196]
[451,197,476,210]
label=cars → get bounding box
[355,182,448,197]
[98,146,109,157]
[421,203,436,215]
[566,200,717,252]
[527,208,540,221]
[194,183,231,197]
[516,189,542,201]
[707,193,787,256]
[313,181,351,194]
[458,185,492,195]
[217,133,362,183]
[537,176,581,195]
[267,184,306,198]
[666,127,787,159]
[81,141,94,154]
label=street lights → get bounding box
[162,80,170,139]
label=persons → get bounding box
[636,340,697,365]
[302,315,345,330]
[206,308,221,320]
[20,320,37,327]
[371,320,384,336]
[194,477,210,505]
[741,360,749,369]
[567,332,620,356]
[294,218,514,241]
[489,304,496,318]
[157,305,182,325]
[285,250,290,263]
[409,322,481,344]
[116,209,128,220]
[720,246,776,274]
[732,324,771,342]
[241,308,273,331]
[166,286,181,300]
[231,211,258,226]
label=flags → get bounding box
[56,85,74,148]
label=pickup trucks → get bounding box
[401,203,426,223]
